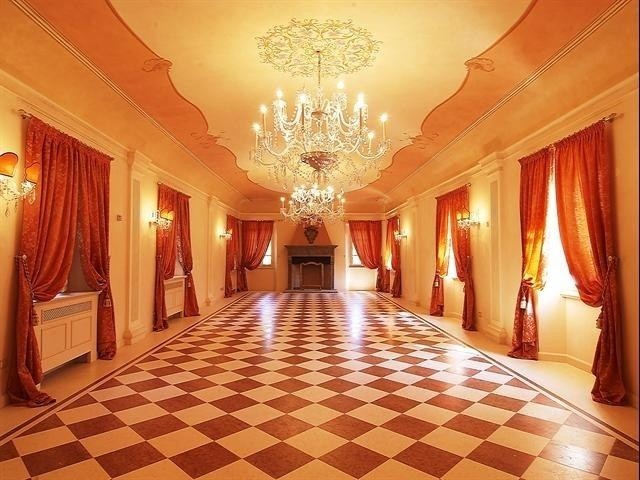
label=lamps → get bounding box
[0,151,42,218]
[248,50,390,227]
